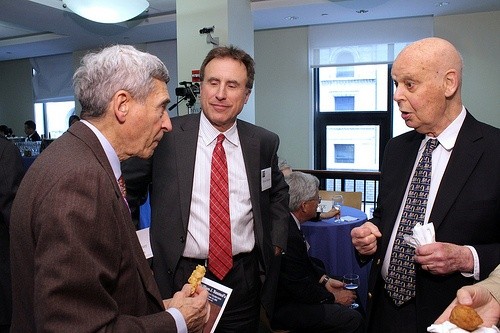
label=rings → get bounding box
[426,264,432,272]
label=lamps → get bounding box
[62,0,149,23]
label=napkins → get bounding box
[317,200,334,213]
[335,216,358,224]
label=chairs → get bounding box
[319,191,362,210]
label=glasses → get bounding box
[306,195,323,204]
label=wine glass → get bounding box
[343,274,360,309]
[331,195,344,223]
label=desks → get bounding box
[303,206,373,310]
[21,157,36,171]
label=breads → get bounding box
[188,264,206,293]
[449,304,483,331]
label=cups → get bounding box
[20,141,41,156]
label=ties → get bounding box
[118,174,132,212]
[385,138,441,306]
[206,133,234,281]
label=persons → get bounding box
[276,158,340,223]
[24,120,40,141]
[69,115,79,128]
[116,45,290,333]
[0,135,25,333]
[349,36,500,333]
[433,262,500,330]
[0,125,13,138]
[8,42,212,333]
[261,171,367,333]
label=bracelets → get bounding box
[321,275,329,286]
